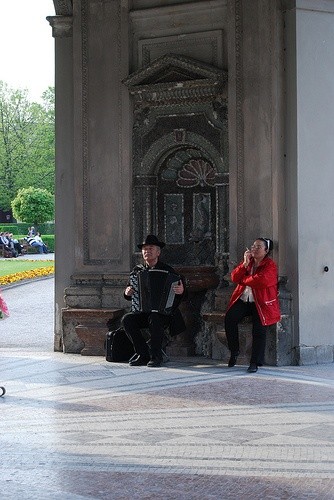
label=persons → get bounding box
[28,227,35,234]
[224,238,281,372]
[0,231,23,257]
[121,235,189,367]
[27,232,49,254]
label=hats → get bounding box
[136,234,166,250]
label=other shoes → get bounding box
[228,352,240,367]
[248,364,258,373]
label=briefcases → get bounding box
[105,327,134,362]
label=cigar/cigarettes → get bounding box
[246,247,249,251]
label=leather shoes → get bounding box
[129,354,148,366]
[147,357,163,367]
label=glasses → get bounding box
[250,244,266,250]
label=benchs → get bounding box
[0,238,48,257]
